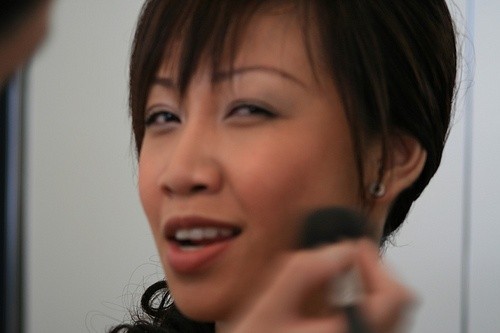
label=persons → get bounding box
[108,0,461,333]
